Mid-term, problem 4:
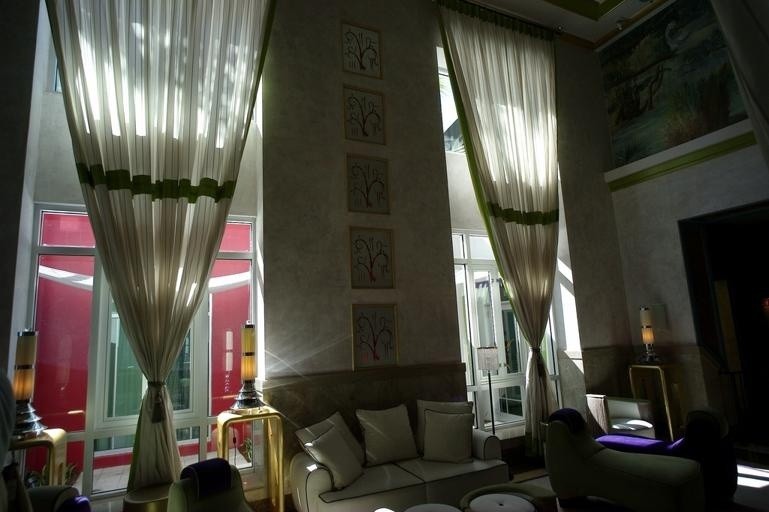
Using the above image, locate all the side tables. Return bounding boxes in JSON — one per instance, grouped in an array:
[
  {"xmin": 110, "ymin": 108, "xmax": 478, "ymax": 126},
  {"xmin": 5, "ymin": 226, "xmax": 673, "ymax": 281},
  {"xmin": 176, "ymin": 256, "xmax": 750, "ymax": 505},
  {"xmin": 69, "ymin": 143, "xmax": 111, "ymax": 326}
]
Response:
[
  {"xmin": 4, "ymin": 428, "xmax": 69, "ymax": 485},
  {"xmin": 217, "ymin": 404, "xmax": 286, "ymax": 512},
  {"xmin": 629, "ymin": 362, "xmax": 681, "ymax": 443}
]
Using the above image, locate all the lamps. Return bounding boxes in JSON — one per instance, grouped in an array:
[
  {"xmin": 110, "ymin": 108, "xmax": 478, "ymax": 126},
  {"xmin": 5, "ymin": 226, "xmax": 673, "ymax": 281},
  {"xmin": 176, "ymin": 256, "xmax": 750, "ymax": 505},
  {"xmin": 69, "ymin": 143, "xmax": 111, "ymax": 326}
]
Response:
[
  {"xmin": 229, "ymin": 324, "xmax": 265, "ymax": 411},
  {"xmin": 636, "ymin": 304, "xmax": 666, "ymax": 364},
  {"xmin": 10, "ymin": 328, "xmax": 48, "ymax": 434},
  {"xmin": 476, "ymin": 346, "xmax": 499, "ymax": 436}
]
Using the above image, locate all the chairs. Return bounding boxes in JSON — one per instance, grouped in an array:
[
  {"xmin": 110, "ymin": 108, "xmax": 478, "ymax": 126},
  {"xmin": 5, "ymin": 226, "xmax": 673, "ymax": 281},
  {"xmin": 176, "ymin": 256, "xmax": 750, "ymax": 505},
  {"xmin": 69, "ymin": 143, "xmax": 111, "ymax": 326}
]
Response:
[{"xmin": 168, "ymin": 458, "xmax": 255, "ymax": 512}]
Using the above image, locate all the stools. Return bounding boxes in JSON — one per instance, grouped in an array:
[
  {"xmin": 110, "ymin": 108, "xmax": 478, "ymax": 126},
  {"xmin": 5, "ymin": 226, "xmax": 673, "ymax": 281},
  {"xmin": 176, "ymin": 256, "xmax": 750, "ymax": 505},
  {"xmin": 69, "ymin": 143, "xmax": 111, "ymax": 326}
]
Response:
[
  {"xmin": 123, "ymin": 482, "xmax": 169, "ymax": 512},
  {"xmin": 403, "ymin": 483, "xmax": 558, "ymax": 512},
  {"xmin": 547, "ymin": 392, "xmax": 738, "ymax": 512}
]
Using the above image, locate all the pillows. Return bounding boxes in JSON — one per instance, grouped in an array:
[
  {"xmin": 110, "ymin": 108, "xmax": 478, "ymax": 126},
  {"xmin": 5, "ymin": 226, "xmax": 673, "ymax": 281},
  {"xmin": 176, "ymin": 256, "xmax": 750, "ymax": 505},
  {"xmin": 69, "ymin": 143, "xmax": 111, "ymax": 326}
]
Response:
[{"xmin": 294, "ymin": 399, "xmax": 474, "ymax": 490}]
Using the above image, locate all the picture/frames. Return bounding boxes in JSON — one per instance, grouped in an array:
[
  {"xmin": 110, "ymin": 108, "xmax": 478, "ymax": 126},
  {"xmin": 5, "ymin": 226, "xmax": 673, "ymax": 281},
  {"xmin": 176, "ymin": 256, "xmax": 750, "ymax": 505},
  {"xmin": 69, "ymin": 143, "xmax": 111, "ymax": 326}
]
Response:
[
  {"xmin": 351, "ymin": 304, "xmax": 400, "ymax": 372},
  {"xmin": 345, "ymin": 152, "xmax": 390, "ymax": 215},
  {"xmin": 340, "ymin": 21, "xmax": 384, "ymax": 81},
  {"xmin": 350, "ymin": 225, "xmax": 396, "ymax": 289},
  {"xmin": 342, "ymin": 84, "xmax": 387, "ymax": 147},
  {"xmin": 595, "ymin": 0, "xmax": 759, "ymax": 191}
]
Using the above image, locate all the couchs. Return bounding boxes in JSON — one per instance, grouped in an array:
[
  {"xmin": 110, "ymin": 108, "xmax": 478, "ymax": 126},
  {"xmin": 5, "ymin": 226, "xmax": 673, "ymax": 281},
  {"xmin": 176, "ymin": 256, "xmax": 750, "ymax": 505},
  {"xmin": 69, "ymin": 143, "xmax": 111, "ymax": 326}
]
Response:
[
  {"xmin": 7, "ymin": 486, "xmax": 91, "ymax": 512},
  {"xmin": 265, "ymin": 362, "xmax": 512, "ymax": 512}
]
